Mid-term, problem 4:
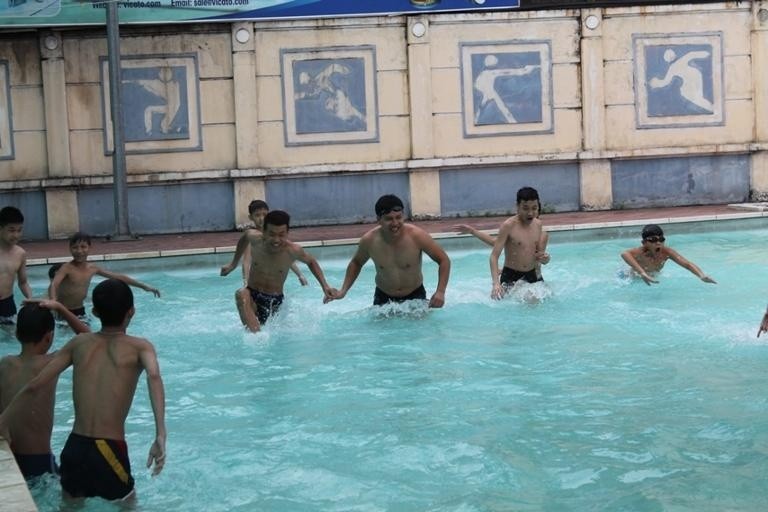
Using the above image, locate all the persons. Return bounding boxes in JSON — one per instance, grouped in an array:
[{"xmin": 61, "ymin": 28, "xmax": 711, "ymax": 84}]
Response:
[
  {"xmin": 241, "ymin": 198, "xmax": 309, "ymax": 289},
  {"xmin": 451, "ymin": 198, "xmax": 551, "ymax": 282},
  {"xmin": 47, "ymin": 262, "xmax": 63, "ymax": 281},
  {"xmin": 47, "ymin": 231, "xmax": 160, "ymax": 336},
  {"xmin": 0, "ymin": 205, "xmax": 33, "ymax": 343},
  {"xmin": 219, "ymin": 209, "xmax": 338, "ymax": 334},
  {"xmin": 0, "ymin": 298, "xmax": 94, "ymax": 487},
  {"xmin": 757, "ymin": 308, "xmax": 768, "ymax": 337},
  {"xmin": 487, "ymin": 186, "xmax": 552, "ymax": 303},
  {"xmin": 321, "ymin": 193, "xmax": 451, "ymax": 319},
  {"xmin": 617, "ymin": 223, "xmax": 718, "ymax": 287},
  {"xmin": 0, "ymin": 279, "xmax": 168, "ymax": 511}
]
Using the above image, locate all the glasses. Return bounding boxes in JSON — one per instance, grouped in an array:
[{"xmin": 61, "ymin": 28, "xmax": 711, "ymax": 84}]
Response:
[{"xmin": 644, "ymin": 236, "xmax": 665, "ymax": 243}]
